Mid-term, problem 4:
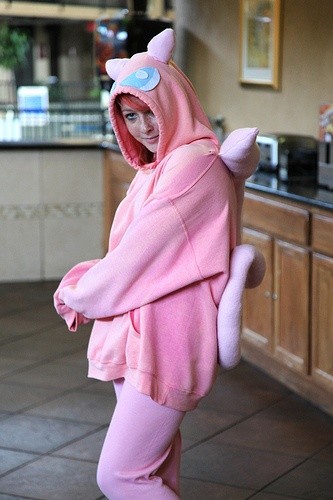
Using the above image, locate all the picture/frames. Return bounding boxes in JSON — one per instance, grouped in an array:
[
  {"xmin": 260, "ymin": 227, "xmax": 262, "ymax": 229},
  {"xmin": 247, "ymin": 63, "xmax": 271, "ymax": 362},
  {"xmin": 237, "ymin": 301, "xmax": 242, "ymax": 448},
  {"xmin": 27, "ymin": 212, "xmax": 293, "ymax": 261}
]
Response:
[{"xmin": 238, "ymin": 0, "xmax": 284, "ymax": 90}]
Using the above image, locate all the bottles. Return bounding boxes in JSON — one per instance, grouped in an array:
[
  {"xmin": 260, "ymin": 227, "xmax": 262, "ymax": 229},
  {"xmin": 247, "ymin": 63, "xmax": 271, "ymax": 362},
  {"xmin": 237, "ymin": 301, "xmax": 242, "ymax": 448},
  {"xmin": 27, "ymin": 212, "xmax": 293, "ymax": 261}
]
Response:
[{"xmin": 18, "ymin": 109, "xmax": 104, "ymax": 139}]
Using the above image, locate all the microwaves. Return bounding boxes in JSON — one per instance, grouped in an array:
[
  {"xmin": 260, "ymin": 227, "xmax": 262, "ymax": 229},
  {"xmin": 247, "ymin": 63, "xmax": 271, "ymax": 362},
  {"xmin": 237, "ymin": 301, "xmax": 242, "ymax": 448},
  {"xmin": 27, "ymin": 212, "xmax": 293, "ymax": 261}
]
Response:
[{"xmin": 256, "ymin": 132, "xmax": 319, "ymax": 183}]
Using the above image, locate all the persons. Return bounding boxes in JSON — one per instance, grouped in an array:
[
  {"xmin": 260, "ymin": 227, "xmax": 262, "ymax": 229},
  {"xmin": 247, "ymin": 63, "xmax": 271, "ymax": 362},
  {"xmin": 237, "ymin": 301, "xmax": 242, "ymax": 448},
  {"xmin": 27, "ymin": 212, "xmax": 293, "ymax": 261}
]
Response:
[{"xmin": 52, "ymin": 52, "xmax": 238, "ymax": 500}]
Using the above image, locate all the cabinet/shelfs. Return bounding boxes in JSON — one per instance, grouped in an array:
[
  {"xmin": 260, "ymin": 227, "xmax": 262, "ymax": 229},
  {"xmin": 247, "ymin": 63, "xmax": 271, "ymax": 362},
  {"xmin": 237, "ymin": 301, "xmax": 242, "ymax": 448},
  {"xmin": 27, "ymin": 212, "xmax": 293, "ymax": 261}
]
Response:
[{"xmin": 103, "ymin": 148, "xmax": 332, "ymax": 416}]
